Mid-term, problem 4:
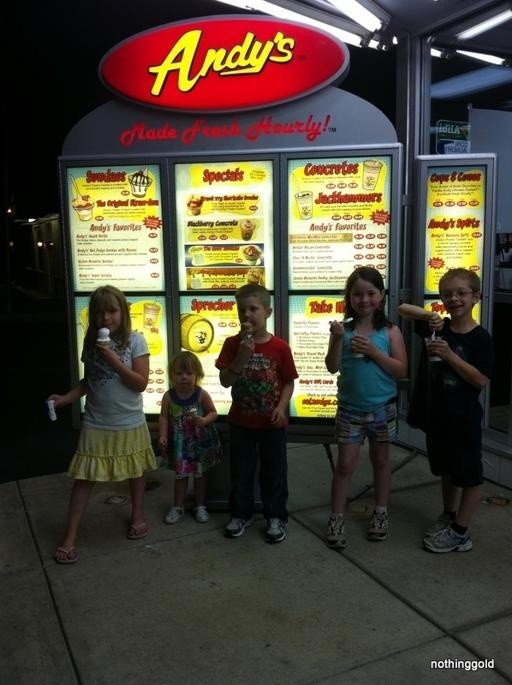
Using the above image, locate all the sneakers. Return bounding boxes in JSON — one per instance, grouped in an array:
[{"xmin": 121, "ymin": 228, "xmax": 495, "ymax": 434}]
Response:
[
  {"xmin": 423, "ymin": 524, "xmax": 474, "ymax": 553},
  {"xmin": 262, "ymin": 516, "xmax": 288, "ymax": 543},
  {"xmin": 324, "ymin": 516, "xmax": 348, "ymax": 548},
  {"xmin": 424, "ymin": 512, "xmax": 457, "ymax": 538},
  {"xmin": 223, "ymin": 514, "xmax": 255, "ymax": 538},
  {"xmin": 365, "ymin": 509, "xmax": 391, "ymax": 540}
]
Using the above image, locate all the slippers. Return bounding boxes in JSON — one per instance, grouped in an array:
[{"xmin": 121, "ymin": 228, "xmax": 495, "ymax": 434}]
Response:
[
  {"xmin": 127, "ymin": 519, "xmax": 151, "ymax": 539},
  {"xmin": 54, "ymin": 545, "xmax": 80, "ymax": 563}
]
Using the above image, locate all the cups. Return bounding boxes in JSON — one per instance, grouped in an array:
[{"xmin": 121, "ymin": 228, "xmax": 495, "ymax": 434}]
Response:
[
  {"xmin": 144, "ymin": 302, "xmax": 161, "ymax": 328},
  {"xmin": 128, "ymin": 176, "xmax": 152, "ymax": 198},
  {"xmin": 423, "ymin": 338, "xmax": 446, "ymax": 362},
  {"xmin": 350, "ymin": 337, "xmax": 366, "ymax": 359},
  {"xmin": 246, "ymin": 270, "xmax": 260, "ymax": 283},
  {"xmin": 295, "ymin": 191, "xmax": 315, "ymax": 219},
  {"xmin": 363, "ymin": 160, "xmax": 382, "ymax": 190},
  {"xmin": 187, "ymin": 201, "xmax": 204, "ymax": 214},
  {"xmin": 239, "ymin": 224, "xmax": 256, "ymax": 240},
  {"xmin": 244, "ymin": 244, "xmax": 262, "ymax": 266},
  {"xmin": 189, "ymin": 246, "xmax": 206, "ymax": 264}
]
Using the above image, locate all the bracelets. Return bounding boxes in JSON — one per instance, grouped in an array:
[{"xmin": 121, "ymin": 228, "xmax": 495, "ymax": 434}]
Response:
[{"xmin": 228, "ymin": 367, "xmax": 243, "ymax": 377}]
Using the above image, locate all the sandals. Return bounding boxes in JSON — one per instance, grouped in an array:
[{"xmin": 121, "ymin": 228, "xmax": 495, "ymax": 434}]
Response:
[
  {"xmin": 165, "ymin": 504, "xmax": 185, "ymax": 525},
  {"xmin": 193, "ymin": 504, "xmax": 210, "ymax": 523}
]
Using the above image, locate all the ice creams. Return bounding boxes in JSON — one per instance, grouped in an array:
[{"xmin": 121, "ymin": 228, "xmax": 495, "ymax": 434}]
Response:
[
  {"xmin": 247, "ymin": 269, "xmax": 260, "ymax": 285},
  {"xmin": 130, "ymin": 169, "xmax": 148, "ymax": 196},
  {"xmin": 241, "ymin": 220, "xmax": 254, "ymax": 240},
  {"xmin": 241, "ymin": 322, "xmax": 253, "ymax": 340},
  {"xmin": 188, "ymin": 198, "xmax": 203, "ymax": 215},
  {"xmin": 96, "ymin": 327, "xmax": 112, "ymax": 346},
  {"xmin": 243, "ymin": 246, "xmax": 260, "ymax": 265}
]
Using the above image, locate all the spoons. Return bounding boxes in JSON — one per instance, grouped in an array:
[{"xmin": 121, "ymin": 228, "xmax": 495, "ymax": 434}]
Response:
[
  {"xmin": 329, "ymin": 317, "xmax": 352, "ymax": 324},
  {"xmin": 431, "ymin": 324, "xmax": 436, "ymax": 341}
]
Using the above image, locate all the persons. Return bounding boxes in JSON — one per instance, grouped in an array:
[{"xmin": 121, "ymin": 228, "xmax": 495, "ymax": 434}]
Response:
[
  {"xmin": 396, "ymin": 265, "xmax": 495, "ymax": 555},
  {"xmin": 46, "ymin": 284, "xmax": 155, "ymax": 564},
  {"xmin": 215, "ymin": 283, "xmax": 298, "ymax": 544},
  {"xmin": 155, "ymin": 351, "xmax": 225, "ymax": 527},
  {"xmin": 323, "ymin": 265, "xmax": 408, "ymax": 551}
]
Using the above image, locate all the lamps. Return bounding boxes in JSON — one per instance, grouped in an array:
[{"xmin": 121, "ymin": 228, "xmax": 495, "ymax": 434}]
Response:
[
  {"xmin": 218, "ymin": 0, "xmax": 399, "ymax": 55},
  {"xmin": 431, "ymin": 5, "xmax": 512, "ymax": 67}
]
